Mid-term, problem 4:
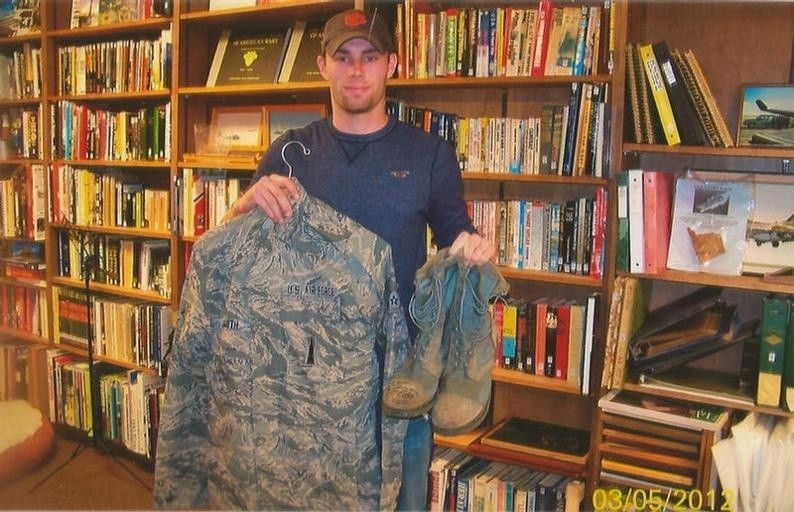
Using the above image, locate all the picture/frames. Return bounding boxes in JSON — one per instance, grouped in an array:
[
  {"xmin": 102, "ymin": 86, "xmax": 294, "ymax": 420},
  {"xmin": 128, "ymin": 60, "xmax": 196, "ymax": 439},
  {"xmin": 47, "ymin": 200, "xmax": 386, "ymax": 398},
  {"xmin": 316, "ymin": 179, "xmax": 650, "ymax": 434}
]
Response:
[
  {"xmin": 680, "ymin": 165, "xmax": 794, "ymax": 274},
  {"xmin": 736, "ymin": 81, "xmax": 788, "ymax": 147},
  {"xmin": 268, "ymin": 102, "xmax": 326, "ymax": 147},
  {"xmin": 209, "ymin": 103, "xmax": 266, "ymax": 154}
]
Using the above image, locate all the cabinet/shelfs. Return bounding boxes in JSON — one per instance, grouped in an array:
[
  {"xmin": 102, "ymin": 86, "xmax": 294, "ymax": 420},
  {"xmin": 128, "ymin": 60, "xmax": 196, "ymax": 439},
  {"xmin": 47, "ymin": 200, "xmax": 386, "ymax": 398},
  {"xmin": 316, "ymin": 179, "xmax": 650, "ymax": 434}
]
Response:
[{"xmin": 1, "ymin": 0, "xmax": 786, "ymax": 510}]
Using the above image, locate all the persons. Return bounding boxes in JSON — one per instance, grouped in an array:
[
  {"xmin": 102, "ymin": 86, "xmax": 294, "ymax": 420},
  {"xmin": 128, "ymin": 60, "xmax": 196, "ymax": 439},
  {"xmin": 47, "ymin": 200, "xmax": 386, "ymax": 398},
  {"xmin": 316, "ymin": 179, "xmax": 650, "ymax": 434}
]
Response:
[{"xmin": 218, "ymin": 8, "xmax": 496, "ymax": 512}]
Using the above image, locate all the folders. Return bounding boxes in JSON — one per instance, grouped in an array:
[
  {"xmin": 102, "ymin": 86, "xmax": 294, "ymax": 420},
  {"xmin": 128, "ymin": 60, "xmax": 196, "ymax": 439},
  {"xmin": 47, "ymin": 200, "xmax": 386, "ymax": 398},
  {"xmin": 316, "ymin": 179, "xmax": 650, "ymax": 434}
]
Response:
[
  {"xmin": 639, "ymin": 44, "xmax": 681, "ymax": 146},
  {"xmin": 628, "ymin": 284, "xmax": 736, "ymax": 367},
  {"xmin": 651, "ymin": 37, "xmax": 700, "ymax": 142},
  {"xmin": 779, "ymin": 296, "xmax": 794, "ymax": 412},
  {"xmin": 756, "ymin": 294, "xmax": 790, "ymax": 407},
  {"xmin": 632, "ymin": 319, "xmax": 760, "ymax": 377},
  {"xmin": 615, "ymin": 168, "xmax": 631, "ymax": 276},
  {"xmin": 642, "ymin": 171, "xmax": 674, "ymax": 275},
  {"xmin": 628, "ymin": 168, "xmax": 645, "ymax": 273}
]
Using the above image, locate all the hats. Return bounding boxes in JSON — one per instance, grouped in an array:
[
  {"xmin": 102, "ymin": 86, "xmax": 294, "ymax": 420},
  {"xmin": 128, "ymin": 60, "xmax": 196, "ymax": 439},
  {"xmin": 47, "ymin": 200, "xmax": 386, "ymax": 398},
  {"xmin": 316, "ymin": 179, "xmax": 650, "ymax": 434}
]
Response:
[{"xmin": 321, "ymin": 10, "xmax": 391, "ymax": 56}]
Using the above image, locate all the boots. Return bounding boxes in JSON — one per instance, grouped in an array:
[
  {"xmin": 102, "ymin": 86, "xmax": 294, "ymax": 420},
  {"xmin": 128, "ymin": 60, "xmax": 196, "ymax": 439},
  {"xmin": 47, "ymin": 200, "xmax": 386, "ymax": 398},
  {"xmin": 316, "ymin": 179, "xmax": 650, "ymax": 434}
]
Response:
[
  {"xmin": 429, "ymin": 260, "xmax": 511, "ymax": 436},
  {"xmin": 382, "ymin": 248, "xmax": 457, "ymax": 418}
]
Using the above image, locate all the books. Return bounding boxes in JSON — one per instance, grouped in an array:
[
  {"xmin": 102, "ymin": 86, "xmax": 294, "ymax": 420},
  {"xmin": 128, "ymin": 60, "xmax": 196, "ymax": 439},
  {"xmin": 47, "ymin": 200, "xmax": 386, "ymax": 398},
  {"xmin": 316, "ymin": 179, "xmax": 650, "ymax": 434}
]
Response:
[
  {"xmin": 425, "ymin": 186, "xmax": 610, "ymax": 280},
  {"xmin": 426, "ymin": 442, "xmax": 587, "ymax": 512},
  {"xmin": 488, "ymin": 290, "xmax": 601, "ymax": 396},
  {"xmin": 624, "ymin": 40, "xmax": 736, "ymax": 148},
  {"xmin": 176, "ymin": 167, "xmax": 252, "ymax": 239},
  {"xmin": 70, "ymin": 0, "xmax": 160, "ymax": 31},
  {"xmin": 49, "ymin": 99, "xmax": 172, "ymax": 162},
  {"xmin": 384, "ymin": 80, "xmax": 613, "ymax": 179},
  {"xmin": 214, "ymin": 25, "xmax": 294, "ymax": 86},
  {"xmin": 0, "ymin": 0, "xmax": 41, "ymax": 38},
  {"xmin": 0, "ymin": 283, "xmax": 50, "ymax": 339},
  {"xmin": 183, "ymin": 147, "xmax": 261, "ymax": 168},
  {"xmin": 596, "ymin": 390, "xmax": 794, "ymax": 512},
  {"xmin": 0, "ymin": 42, "xmax": 43, "ymax": 101},
  {"xmin": 600, "ymin": 275, "xmax": 794, "ymax": 415},
  {"xmin": 0, "ymin": 342, "xmax": 165, "ymax": 462},
  {"xmin": 288, "ymin": 19, "xmax": 327, "ymax": 82},
  {"xmin": 0, "ymin": 104, "xmax": 44, "ymax": 162},
  {"xmin": 47, "ymin": 163, "xmax": 173, "ymax": 235},
  {"xmin": 616, "ymin": 168, "xmax": 675, "ymax": 274},
  {"xmin": 468, "ymin": 413, "xmax": 591, "ymax": 473},
  {"xmin": 393, "ymin": 0, "xmax": 616, "ymax": 79},
  {"xmin": 1, "ymin": 240, "xmax": 47, "ymax": 287},
  {"xmin": 56, "ymin": 228, "xmax": 174, "ymax": 301},
  {"xmin": 184, "ymin": 241, "xmax": 194, "ymax": 272},
  {"xmin": 56, "ymin": 29, "xmax": 174, "ymax": 95},
  {"xmin": 51, "ymin": 284, "xmax": 174, "ymax": 378},
  {"xmin": 0, "ymin": 163, "xmax": 46, "ymax": 241}
]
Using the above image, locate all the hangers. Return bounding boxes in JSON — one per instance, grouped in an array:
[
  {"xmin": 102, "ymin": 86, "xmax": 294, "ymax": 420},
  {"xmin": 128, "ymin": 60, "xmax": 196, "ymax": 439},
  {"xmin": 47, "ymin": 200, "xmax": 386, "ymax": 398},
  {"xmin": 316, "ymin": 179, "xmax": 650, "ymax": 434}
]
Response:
[{"xmin": 264, "ymin": 140, "xmax": 312, "ymax": 202}]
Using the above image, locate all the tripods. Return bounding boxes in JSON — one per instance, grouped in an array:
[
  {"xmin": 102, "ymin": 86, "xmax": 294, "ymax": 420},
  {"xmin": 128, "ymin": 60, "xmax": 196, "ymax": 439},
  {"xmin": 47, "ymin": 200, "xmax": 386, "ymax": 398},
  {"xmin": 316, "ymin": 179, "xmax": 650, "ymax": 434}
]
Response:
[{"xmin": 27, "ymin": 210, "xmax": 153, "ymax": 495}]
